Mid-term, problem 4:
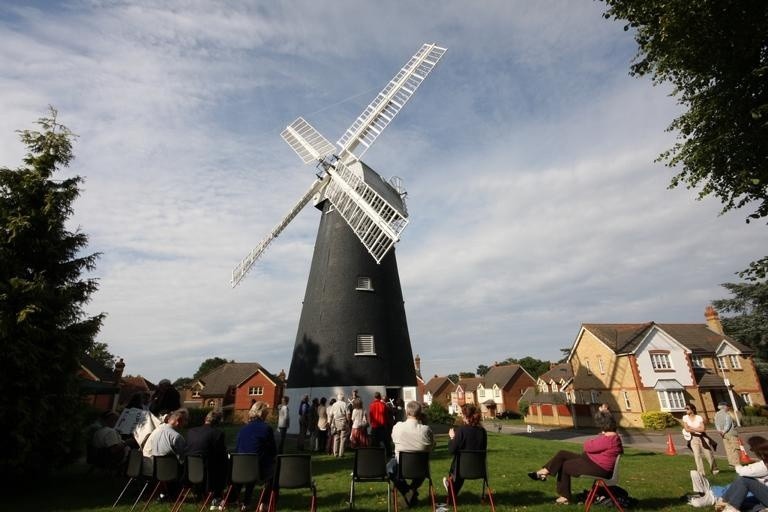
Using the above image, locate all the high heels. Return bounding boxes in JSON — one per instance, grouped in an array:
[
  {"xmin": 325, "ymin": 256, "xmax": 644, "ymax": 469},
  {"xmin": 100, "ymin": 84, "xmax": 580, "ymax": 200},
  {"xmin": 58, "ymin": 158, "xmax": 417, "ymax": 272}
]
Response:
[
  {"xmin": 664, "ymin": 434, "xmax": 678, "ymax": 458},
  {"xmin": 734, "ymin": 438, "xmax": 756, "ymax": 468}
]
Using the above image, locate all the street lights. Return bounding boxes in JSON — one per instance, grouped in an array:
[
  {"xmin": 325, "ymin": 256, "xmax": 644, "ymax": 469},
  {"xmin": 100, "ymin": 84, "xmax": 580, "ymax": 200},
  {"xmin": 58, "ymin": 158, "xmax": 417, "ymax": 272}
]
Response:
[{"xmin": 361, "ymin": 416, "xmax": 368, "ymax": 427}]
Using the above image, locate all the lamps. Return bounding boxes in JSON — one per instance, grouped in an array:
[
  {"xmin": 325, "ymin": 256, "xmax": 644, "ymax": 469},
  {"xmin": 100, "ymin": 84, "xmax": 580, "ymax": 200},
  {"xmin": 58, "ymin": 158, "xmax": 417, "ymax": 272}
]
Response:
[
  {"xmin": 110, "ymin": 449, "xmax": 317, "ymax": 511},
  {"xmin": 348, "ymin": 446, "xmax": 495, "ymax": 511},
  {"xmin": 579, "ymin": 455, "xmax": 622, "ymax": 509}
]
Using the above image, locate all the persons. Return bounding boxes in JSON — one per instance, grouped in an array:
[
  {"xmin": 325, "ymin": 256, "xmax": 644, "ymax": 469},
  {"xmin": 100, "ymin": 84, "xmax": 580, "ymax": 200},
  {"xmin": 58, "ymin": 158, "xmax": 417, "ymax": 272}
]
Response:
[
  {"xmin": 598, "ymin": 403, "xmax": 613, "ymax": 417},
  {"xmin": 94, "ymin": 411, "xmax": 134, "ymax": 464},
  {"xmin": 713, "ymin": 435, "xmax": 767, "ymax": 512},
  {"xmin": 443, "ymin": 403, "xmax": 487, "ymax": 509},
  {"xmin": 386, "ymin": 400, "xmax": 436, "ymax": 509},
  {"xmin": 680, "ymin": 404, "xmax": 719, "ymax": 478},
  {"xmin": 276, "ymin": 396, "xmax": 292, "ymax": 454},
  {"xmin": 713, "ymin": 401, "xmax": 742, "ymax": 472},
  {"xmin": 150, "ymin": 409, "xmax": 189, "ymax": 502},
  {"xmin": 233, "ymin": 401, "xmax": 277, "ymax": 499},
  {"xmin": 298, "ymin": 388, "xmax": 406, "ymax": 458},
  {"xmin": 527, "ymin": 412, "xmax": 624, "ymax": 506},
  {"xmin": 185, "ymin": 410, "xmax": 233, "ymax": 509}
]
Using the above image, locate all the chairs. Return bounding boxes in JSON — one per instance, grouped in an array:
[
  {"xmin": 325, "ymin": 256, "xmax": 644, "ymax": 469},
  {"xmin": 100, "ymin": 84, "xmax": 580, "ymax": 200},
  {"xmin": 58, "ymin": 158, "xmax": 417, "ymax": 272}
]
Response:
[
  {"xmin": 528, "ymin": 472, "xmax": 546, "ymax": 481},
  {"xmin": 210, "ymin": 497, "xmax": 221, "ymax": 510},
  {"xmin": 219, "ymin": 499, "xmax": 228, "ymax": 510},
  {"xmin": 443, "ymin": 477, "xmax": 449, "ymax": 490}
]
